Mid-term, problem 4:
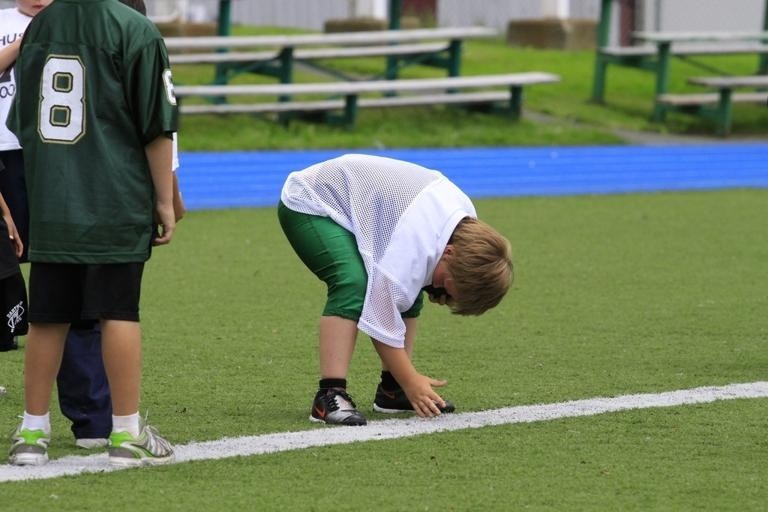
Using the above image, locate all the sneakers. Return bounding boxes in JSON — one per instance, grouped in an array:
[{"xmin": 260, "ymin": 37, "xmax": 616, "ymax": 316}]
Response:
[
  {"xmin": 309, "ymin": 386, "xmax": 367, "ymax": 427},
  {"xmin": 75, "ymin": 434, "xmax": 111, "ymax": 449},
  {"xmin": 7, "ymin": 427, "xmax": 52, "ymax": 466},
  {"xmin": 107, "ymin": 423, "xmax": 178, "ymax": 465},
  {"xmin": 371, "ymin": 382, "xmax": 455, "ymax": 414}
]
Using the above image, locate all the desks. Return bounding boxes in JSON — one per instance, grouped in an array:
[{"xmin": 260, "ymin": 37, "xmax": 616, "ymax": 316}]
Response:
[
  {"xmin": 632, "ymin": 31, "xmax": 767, "ymax": 126},
  {"xmin": 162, "ymin": 26, "xmax": 500, "ymax": 129}
]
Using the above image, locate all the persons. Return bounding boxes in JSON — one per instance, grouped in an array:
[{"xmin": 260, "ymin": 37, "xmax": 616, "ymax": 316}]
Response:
[
  {"xmin": 277, "ymin": 153, "xmax": 515, "ymax": 426},
  {"xmin": 0, "ymin": 1, "xmax": 186, "ymax": 467}
]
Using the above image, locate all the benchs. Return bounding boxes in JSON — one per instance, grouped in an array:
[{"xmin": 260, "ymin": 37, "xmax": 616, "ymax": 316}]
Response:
[
  {"xmin": 590, "ymin": 42, "xmax": 767, "ymax": 124},
  {"xmin": 161, "ymin": 42, "xmax": 562, "ymax": 130}
]
